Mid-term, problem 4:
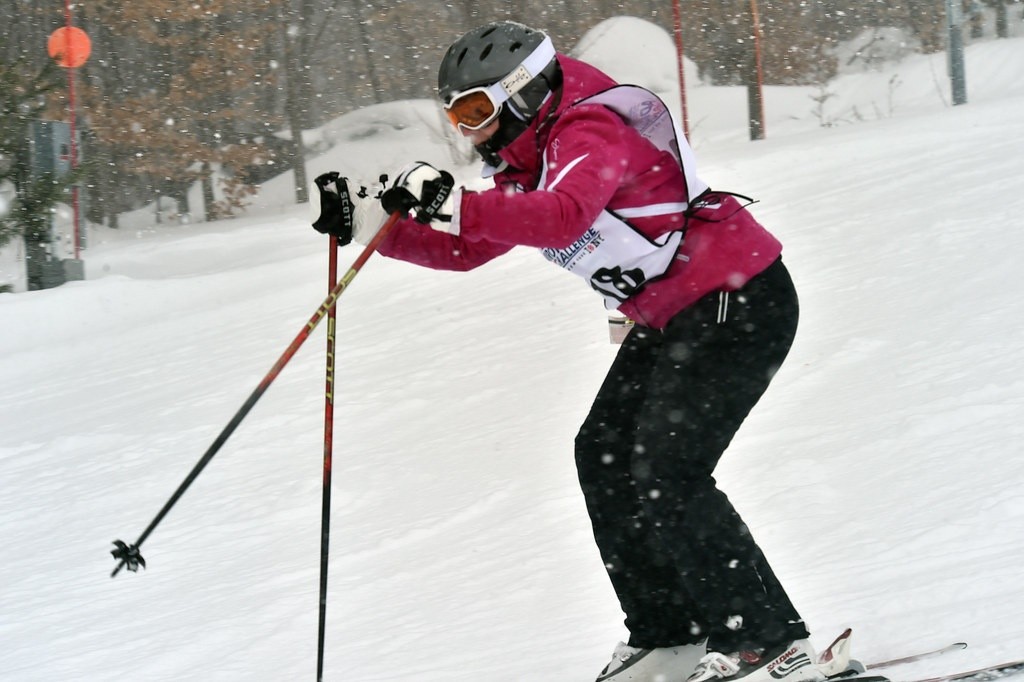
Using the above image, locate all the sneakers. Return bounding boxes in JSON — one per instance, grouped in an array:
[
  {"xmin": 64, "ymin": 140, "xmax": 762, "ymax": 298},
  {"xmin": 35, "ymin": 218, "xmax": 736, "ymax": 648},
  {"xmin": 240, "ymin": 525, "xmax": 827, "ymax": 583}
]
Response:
[
  {"xmin": 687, "ymin": 639, "xmax": 826, "ymax": 682},
  {"xmin": 595, "ymin": 634, "xmax": 708, "ymax": 681}
]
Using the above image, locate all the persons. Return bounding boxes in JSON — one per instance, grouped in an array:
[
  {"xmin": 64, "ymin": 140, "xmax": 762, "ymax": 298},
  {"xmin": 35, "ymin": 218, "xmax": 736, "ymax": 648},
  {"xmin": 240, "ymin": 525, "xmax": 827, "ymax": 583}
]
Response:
[{"xmin": 314, "ymin": 19, "xmax": 823, "ymax": 682}]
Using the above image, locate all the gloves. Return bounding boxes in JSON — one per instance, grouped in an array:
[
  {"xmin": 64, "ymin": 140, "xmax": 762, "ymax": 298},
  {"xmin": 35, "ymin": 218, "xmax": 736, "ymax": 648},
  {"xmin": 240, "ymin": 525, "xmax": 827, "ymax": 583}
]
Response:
[
  {"xmin": 381, "ymin": 160, "xmax": 465, "ymax": 238},
  {"xmin": 308, "ymin": 170, "xmax": 391, "ymax": 247}
]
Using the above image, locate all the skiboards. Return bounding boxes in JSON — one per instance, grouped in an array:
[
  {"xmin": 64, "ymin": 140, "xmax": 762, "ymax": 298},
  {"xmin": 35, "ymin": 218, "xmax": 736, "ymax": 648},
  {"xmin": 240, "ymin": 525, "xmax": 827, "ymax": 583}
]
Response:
[{"xmin": 594, "ymin": 640, "xmax": 1023, "ymax": 682}]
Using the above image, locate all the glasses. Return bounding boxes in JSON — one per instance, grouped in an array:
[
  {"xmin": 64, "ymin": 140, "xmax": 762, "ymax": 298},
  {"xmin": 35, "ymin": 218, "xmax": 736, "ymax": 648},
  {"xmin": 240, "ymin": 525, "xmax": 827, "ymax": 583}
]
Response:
[{"xmin": 443, "ymin": 36, "xmax": 556, "ymax": 138}]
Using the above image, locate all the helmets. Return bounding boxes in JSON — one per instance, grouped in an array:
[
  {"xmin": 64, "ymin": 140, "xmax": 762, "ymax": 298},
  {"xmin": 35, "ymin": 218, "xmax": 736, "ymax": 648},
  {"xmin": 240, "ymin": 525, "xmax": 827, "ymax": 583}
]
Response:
[{"xmin": 436, "ymin": 19, "xmax": 564, "ymax": 173}]
